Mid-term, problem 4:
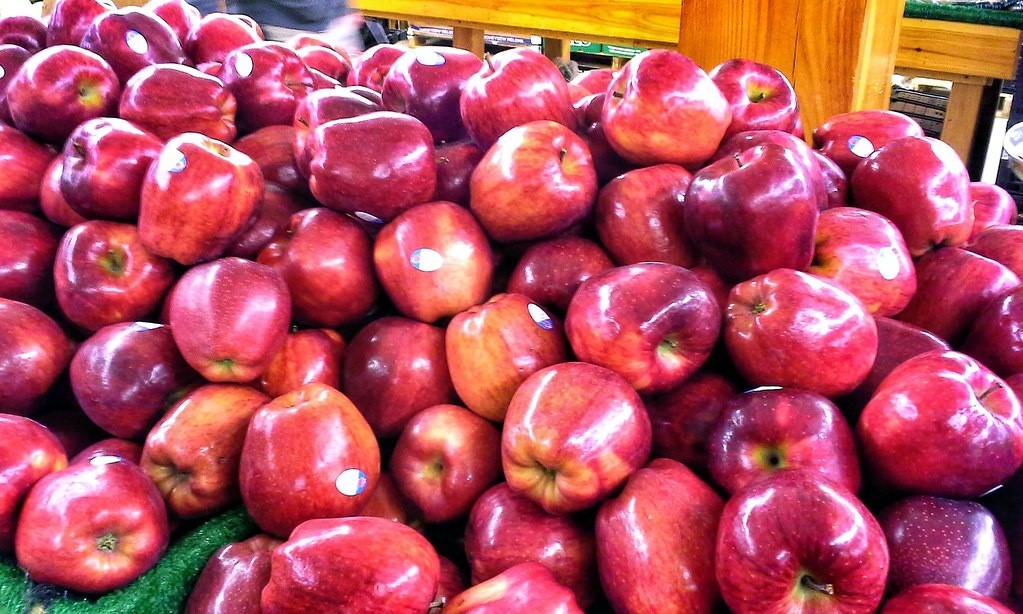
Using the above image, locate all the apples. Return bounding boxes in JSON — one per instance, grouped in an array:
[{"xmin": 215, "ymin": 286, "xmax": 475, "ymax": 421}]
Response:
[{"xmin": 0, "ymin": 0, "xmax": 1023, "ymax": 614}]
